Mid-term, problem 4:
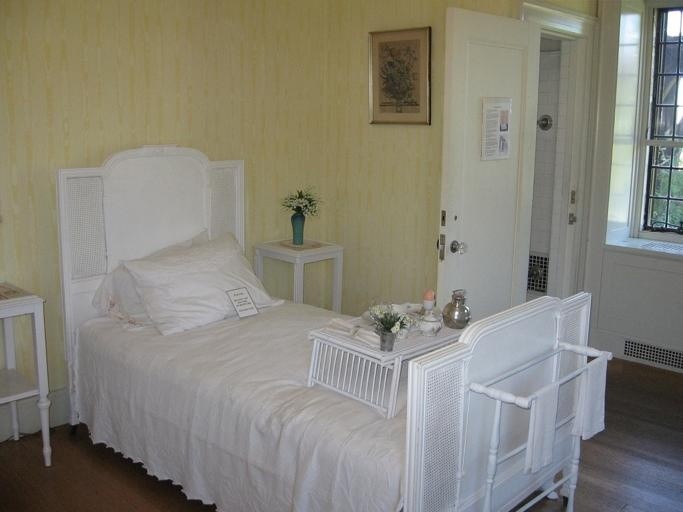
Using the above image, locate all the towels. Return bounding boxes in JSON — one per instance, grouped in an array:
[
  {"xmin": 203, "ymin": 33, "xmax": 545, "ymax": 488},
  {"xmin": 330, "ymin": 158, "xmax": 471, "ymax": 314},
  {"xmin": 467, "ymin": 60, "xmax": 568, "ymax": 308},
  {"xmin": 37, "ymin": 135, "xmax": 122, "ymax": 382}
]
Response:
[
  {"xmin": 569, "ymin": 354, "xmax": 606, "ymax": 440},
  {"xmin": 523, "ymin": 382, "xmax": 559, "ymax": 474}
]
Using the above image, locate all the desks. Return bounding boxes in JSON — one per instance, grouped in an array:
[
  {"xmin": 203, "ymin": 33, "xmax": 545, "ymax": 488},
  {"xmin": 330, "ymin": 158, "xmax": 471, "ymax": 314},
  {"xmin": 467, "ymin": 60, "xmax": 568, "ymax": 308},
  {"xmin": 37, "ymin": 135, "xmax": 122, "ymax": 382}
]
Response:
[{"xmin": 306, "ymin": 304, "xmax": 469, "ymax": 418}]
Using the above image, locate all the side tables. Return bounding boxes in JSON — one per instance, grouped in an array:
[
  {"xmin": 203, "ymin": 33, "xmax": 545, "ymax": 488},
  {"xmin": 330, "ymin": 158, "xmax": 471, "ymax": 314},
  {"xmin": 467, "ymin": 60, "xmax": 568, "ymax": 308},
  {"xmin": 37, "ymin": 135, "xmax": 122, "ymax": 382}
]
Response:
[
  {"xmin": 0, "ymin": 282, "xmax": 52, "ymax": 469},
  {"xmin": 255, "ymin": 238, "xmax": 345, "ymax": 308}
]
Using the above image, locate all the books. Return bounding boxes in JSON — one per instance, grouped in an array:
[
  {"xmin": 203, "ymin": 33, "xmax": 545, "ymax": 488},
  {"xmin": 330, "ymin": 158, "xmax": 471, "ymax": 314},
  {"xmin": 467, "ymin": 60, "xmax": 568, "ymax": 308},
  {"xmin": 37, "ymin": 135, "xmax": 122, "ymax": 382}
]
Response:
[{"xmin": 224, "ymin": 286, "xmax": 260, "ymax": 322}]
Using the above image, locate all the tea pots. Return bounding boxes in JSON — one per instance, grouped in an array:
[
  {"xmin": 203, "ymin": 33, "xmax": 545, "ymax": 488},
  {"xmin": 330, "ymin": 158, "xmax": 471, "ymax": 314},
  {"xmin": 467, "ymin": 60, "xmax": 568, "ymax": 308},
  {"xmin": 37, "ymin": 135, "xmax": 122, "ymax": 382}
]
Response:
[
  {"xmin": 443, "ymin": 289, "xmax": 472, "ymax": 329},
  {"xmin": 414, "ymin": 310, "xmax": 443, "ymax": 337}
]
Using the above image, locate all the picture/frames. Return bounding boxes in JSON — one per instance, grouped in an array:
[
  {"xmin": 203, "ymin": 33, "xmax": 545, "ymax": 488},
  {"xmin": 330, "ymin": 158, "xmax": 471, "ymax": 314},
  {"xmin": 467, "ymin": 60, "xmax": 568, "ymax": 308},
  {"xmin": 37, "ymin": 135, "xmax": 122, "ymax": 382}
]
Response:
[{"xmin": 366, "ymin": 26, "xmax": 432, "ymax": 125}]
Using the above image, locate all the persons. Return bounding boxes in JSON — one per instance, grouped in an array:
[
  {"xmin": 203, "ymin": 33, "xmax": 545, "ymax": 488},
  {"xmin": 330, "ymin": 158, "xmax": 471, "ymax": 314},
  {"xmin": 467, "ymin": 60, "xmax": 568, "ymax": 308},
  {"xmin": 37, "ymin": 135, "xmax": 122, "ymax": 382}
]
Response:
[
  {"xmin": 502, "ymin": 139, "xmax": 508, "ymax": 155},
  {"xmin": 499, "ymin": 111, "xmax": 508, "ymax": 131}
]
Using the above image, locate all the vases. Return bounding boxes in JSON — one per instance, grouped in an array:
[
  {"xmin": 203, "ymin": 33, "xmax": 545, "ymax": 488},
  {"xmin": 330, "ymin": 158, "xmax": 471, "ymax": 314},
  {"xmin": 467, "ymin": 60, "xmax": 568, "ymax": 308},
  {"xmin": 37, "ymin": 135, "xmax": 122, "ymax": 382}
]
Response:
[
  {"xmin": 380, "ymin": 330, "xmax": 394, "ymax": 350},
  {"xmin": 291, "ymin": 211, "xmax": 305, "ymax": 246}
]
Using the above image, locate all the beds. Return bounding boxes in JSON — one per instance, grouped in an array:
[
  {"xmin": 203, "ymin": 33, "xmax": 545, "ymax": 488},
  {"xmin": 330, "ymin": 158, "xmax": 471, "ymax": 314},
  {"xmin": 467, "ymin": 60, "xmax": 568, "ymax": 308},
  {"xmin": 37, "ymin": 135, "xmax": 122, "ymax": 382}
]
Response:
[{"xmin": 58, "ymin": 144, "xmax": 592, "ymax": 512}]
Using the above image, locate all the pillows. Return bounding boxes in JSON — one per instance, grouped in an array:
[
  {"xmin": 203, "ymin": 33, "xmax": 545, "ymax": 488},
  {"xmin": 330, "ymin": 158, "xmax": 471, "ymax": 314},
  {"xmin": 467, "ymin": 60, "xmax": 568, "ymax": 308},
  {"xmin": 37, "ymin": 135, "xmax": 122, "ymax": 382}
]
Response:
[
  {"xmin": 98, "ymin": 230, "xmax": 212, "ymax": 328},
  {"xmin": 123, "ymin": 232, "xmax": 276, "ymax": 338}
]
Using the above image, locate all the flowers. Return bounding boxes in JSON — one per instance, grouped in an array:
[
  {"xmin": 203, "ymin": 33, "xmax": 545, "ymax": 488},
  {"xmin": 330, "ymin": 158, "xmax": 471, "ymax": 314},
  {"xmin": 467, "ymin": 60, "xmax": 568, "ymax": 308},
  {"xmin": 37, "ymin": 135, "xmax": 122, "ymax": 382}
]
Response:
[
  {"xmin": 283, "ymin": 183, "xmax": 323, "ymax": 215},
  {"xmin": 364, "ymin": 300, "xmax": 419, "ymax": 334}
]
